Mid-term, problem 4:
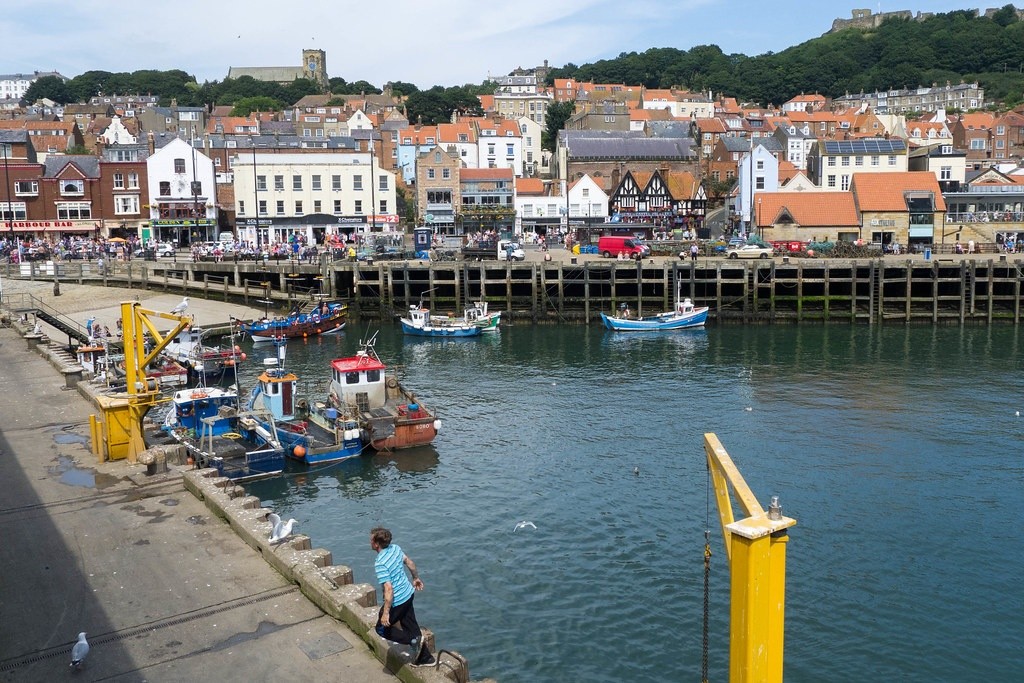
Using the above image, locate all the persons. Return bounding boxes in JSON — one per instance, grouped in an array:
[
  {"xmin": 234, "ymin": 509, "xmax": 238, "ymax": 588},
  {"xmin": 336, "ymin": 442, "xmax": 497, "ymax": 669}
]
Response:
[
  {"xmin": 1003, "ymin": 239, "xmax": 1024, "ymax": 254},
  {"xmin": 518, "ymin": 229, "xmax": 747, "ymax": 260},
  {"xmin": 467, "ymin": 229, "xmax": 502, "ymax": 248},
  {"xmin": 954, "ymin": 240, "xmax": 982, "ymax": 254},
  {"xmin": 191, "ymin": 232, "xmax": 404, "ymax": 262},
  {"xmin": 968, "ymin": 209, "xmax": 1020, "ymax": 222},
  {"xmin": 370, "ymin": 528, "xmax": 436, "ymax": 667},
  {"xmin": 0, "ymin": 233, "xmax": 173, "ymax": 269},
  {"xmin": 87, "ymin": 316, "xmax": 112, "ymax": 338},
  {"xmin": 886, "ymin": 241, "xmax": 900, "ymax": 255},
  {"xmin": 322, "ymin": 302, "xmax": 329, "ymax": 316}
]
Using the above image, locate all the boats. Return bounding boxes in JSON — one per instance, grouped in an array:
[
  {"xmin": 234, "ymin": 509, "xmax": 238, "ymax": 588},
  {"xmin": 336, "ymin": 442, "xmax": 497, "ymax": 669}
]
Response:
[
  {"xmin": 234, "ymin": 293, "xmax": 349, "ymax": 344},
  {"xmin": 237, "ymin": 316, "xmax": 366, "ymax": 467},
  {"xmin": 75, "ymin": 309, "xmax": 247, "ymax": 396},
  {"xmin": 161, "ymin": 322, "xmax": 288, "ymax": 486},
  {"xmin": 399, "ymin": 287, "xmax": 503, "ymax": 338},
  {"xmin": 311, "ymin": 318, "xmax": 442, "ymax": 454},
  {"xmin": 599, "ymin": 272, "xmax": 710, "ymax": 333}
]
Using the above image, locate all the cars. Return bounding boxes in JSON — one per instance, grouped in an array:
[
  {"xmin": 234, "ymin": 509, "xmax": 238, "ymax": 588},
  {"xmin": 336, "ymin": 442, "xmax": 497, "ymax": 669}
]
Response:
[
  {"xmin": 134, "ymin": 243, "xmax": 176, "ymax": 258},
  {"xmin": 726, "ymin": 243, "xmax": 774, "ymax": 260},
  {"xmin": 198, "ymin": 231, "xmax": 256, "ymax": 262},
  {"xmin": 61, "ymin": 245, "xmax": 100, "ymax": 260}
]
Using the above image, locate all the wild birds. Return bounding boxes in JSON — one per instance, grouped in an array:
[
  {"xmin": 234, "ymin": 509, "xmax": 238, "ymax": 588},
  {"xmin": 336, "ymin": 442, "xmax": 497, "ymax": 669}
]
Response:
[
  {"xmin": 68, "ymin": 631, "xmax": 90, "ymax": 667},
  {"xmin": 170, "ymin": 297, "xmax": 190, "ymax": 315},
  {"xmin": 513, "ymin": 520, "xmax": 536, "ymax": 533},
  {"xmin": 17, "ymin": 314, "xmax": 26, "ymax": 323},
  {"xmin": 745, "ymin": 406, "xmax": 752, "ymax": 412},
  {"xmin": 265, "ymin": 511, "xmax": 298, "ymax": 545},
  {"xmin": 1015, "ymin": 411, "xmax": 1020, "ymax": 417},
  {"xmin": 33, "ymin": 324, "xmax": 40, "ymax": 335},
  {"xmin": 634, "ymin": 466, "xmax": 639, "ymax": 475}
]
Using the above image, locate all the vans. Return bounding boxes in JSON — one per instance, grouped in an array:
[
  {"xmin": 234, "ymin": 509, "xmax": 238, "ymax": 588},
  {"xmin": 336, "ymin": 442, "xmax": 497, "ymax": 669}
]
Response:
[{"xmin": 598, "ymin": 236, "xmax": 650, "ymax": 260}]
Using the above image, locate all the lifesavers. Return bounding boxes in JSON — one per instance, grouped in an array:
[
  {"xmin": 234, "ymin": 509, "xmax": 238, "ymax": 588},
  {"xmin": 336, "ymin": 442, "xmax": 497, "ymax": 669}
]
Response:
[
  {"xmin": 190, "ymin": 393, "xmax": 207, "ymax": 399},
  {"xmin": 334, "ymin": 308, "xmax": 340, "ymax": 317},
  {"xmin": 312, "ymin": 314, "xmax": 320, "ymax": 323}
]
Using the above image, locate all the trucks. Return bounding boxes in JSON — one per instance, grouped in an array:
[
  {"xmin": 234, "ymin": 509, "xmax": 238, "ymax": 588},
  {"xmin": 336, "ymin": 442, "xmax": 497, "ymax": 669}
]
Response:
[{"xmin": 461, "ymin": 240, "xmax": 526, "ymax": 261}]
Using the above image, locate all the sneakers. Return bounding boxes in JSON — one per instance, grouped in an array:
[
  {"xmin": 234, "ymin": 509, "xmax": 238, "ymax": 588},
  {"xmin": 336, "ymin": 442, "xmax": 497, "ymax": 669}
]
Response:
[
  {"xmin": 410, "ymin": 635, "xmax": 424, "ymax": 663},
  {"xmin": 410, "ymin": 656, "xmax": 437, "ymax": 668}
]
